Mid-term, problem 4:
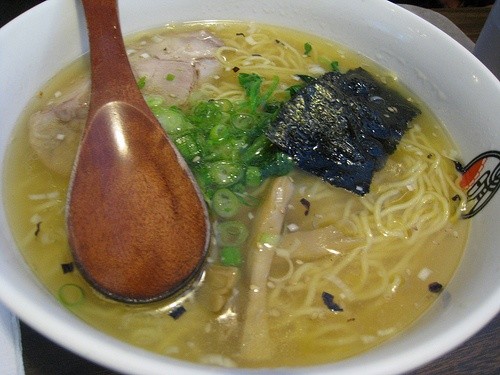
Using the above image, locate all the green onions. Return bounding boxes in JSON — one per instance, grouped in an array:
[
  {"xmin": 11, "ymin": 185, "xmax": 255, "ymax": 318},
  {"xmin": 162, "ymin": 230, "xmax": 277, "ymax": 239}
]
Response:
[{"xmin": 60, "ymin": 69, "xmax": 301, "ymax": 306}]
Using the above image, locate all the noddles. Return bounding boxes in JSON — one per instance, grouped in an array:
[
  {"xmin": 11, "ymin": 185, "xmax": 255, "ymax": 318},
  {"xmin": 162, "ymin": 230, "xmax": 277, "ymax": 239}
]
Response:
[{"xmin": 175, "ymin": 31, "xmax": 469, "ymax": 344}]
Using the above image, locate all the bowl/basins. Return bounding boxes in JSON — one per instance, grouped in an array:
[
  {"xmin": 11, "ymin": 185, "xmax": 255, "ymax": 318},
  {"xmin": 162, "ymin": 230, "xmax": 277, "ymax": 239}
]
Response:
[{"xmin": 0, "ymin": 0, "xmax": 499, "ymax": 375}]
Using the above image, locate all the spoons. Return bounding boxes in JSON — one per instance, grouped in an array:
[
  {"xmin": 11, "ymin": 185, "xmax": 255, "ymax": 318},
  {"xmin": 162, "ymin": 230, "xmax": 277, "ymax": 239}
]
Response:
[{"xmin": 65, "ymin": 0, "xmax": 211, "ymax": 303}]
